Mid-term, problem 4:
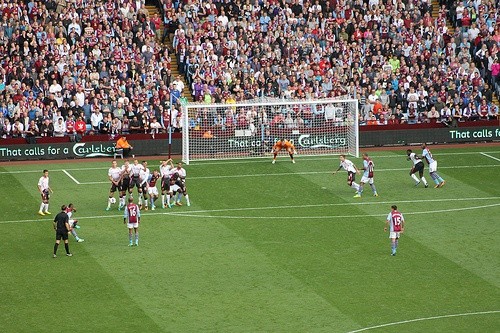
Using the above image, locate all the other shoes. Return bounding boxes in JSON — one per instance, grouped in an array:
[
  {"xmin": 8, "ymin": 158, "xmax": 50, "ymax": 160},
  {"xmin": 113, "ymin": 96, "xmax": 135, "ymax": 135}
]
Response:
[
  {"xmin": 135, "ymin": 243, "xmax": 139, "ymax": 246},
  {"xmin": 425, "ymin": 183, "xmax": 429, "ymax": 188},
  {"xmin": 139, "ymin": 205, "xmax": 142, "ymax": 210},
  {"xmin": 145, "ymin": 206, "xmax": 149, "ymax": 211},
  {"xmin": 53, "ymin": 254, "xmax": 57, "ymax": 258},
  {"xmin": 272, "ymin": 159, "xmax": 276, "ymax": 164},
  {"xmin": 440, "ymin": 180, "xmax": 446, "ymax": 188},
  {"xmin": 127, "ymin": 243, "xmax": 133, "ymax": 247},
  {"xmin": 38, "ymin": 211, "xmax": 45, "ymax": 216},
  {"xmin": 434, "ymin": 183, "xmax": 440, "ymax": 188},
  {"xmin": 150, "ymin": 203, "xmax": 156, "ymax": 210},
  {"xmin": 162, "ymin": 202, "xmax": 190, "ymax": 208},
  {"xmin": 391, "ymin": 248, "xmax": 397, "ymax": 255},
  {"xmin": 291, "ymin": 160, "xmax": 295, "ymax": 163},
  {"xmin": 77, "ymin": 239, "xmax": 84, "ymax": 243},
  {"xmin": 45, "ymin": 211, "xmax": 52, "ymax": 214},
  {"xmin": 66, "ymin": 254, "xmax": 72, "ymax": 256},
  {"xmin": 373, "ymin": 193, "xmax": 378, "ymax": 196},
  {"xmin": 355, "ymin": 190, "xmax": 363, "ymax": 193},
  {"xmin": 106, "ymin": 207, "xmax": 111, "ymax": 211},
  {"xmin": 415, "ymin": 180, "xmax": 420, "ymax": 186},
  {"xmin": 353, "ymin": 194, "xmax": 361, "ymax": 198}
]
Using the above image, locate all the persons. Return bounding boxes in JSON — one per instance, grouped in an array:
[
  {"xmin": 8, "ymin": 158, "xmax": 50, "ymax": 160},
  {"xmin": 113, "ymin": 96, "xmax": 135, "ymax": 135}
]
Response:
[
  {"xmin": 124, "ymin": 197, "xmax": 141, "ymax": 247},
  {"xmin": 52, "ymin": 205, "xmax": 73, "ymax": 258},
  {"xmin": 66, "ymin": 203, "xmax": 84, "ymax": 243},
  {"xmin": 0, "ymin": 0, "xmax": 500, "ymax": 216},
  {"xmin": 384, "ymin": 205, "xmax": 405, "ymax": 256}
]
